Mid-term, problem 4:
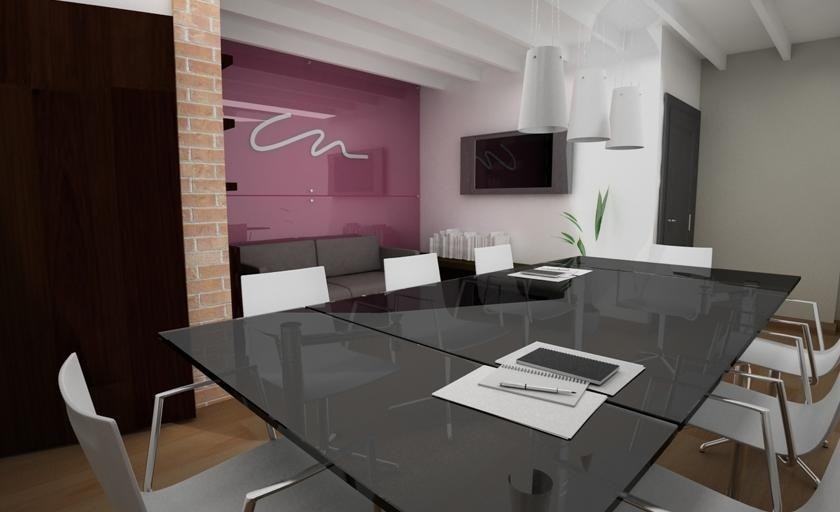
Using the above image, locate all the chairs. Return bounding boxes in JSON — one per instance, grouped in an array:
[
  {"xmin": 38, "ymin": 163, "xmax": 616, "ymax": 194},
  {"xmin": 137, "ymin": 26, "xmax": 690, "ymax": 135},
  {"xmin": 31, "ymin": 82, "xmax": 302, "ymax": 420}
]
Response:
[
  {"xmin": 240, "ymin": 264, "xmax": 335, "ymax": 441},
  {"xmin": 616, "ymin": 440, "xmax": 840, "ymax": 511},
  {"xmin": 56, "ymin": 350, "xmax": 372, "ymax": 508},
  {"xmin": 697, "ymin": 326, "xmax": 837, "ymax": 499},
  {"xmin": 735, "ymin": 284, "xmax": 838, "ymax": 398},
  {"xmin": 379, "ymin": 251, "xmax": 444, "ymax": 291},
  {"xmin": 647, "ymin": 243, "xmax": 714, "ymax": 277},
  {"xmin": 472, "ymin": 242, "xmax": 517, "ymax": 277}
]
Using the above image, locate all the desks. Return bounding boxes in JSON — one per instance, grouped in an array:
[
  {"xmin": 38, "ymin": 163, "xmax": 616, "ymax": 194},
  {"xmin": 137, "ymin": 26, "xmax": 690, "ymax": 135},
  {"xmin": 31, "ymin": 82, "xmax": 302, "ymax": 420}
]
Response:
[{"xmin": 168, "ymin": 255, "xmax": 803, "ymax": 511}]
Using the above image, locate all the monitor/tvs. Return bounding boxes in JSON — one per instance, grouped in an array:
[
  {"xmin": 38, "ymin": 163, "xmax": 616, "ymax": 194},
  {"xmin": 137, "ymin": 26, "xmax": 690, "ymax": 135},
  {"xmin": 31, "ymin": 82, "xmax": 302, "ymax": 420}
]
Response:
[
  {"xmin": 460, "ymin": 130, "xmax": 572, "ymax": 194},
  {"xmin": 326, "ymin": 150, "xmax": 386, "ymax": 195}
]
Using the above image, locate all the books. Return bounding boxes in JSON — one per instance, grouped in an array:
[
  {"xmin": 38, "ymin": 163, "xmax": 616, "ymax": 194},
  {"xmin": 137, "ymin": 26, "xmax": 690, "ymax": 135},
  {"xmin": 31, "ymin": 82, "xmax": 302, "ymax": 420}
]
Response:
[
  {"xmin": 519, "ymin": 268, "xmax": 566, "ymax": 278},
  {"xmin": 477, "ymin": 364, "xmax": 590, "ymax": 407},
  {"xmin": 516, "ymin": 348, "xmax": 619, "ymax": 385}
]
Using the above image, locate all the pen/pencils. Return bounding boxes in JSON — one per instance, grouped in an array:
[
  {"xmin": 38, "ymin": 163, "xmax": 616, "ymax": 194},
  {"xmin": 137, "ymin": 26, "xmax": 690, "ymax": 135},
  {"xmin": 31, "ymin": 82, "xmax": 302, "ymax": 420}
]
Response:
[{"xmin": 499, "ymin": 381, "xmax": 584, "ymax": 398}]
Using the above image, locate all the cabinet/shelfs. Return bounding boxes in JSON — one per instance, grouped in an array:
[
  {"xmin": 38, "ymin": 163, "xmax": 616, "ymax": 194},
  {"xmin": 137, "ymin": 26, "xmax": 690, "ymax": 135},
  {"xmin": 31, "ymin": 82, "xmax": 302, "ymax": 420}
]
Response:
[{"xmin": 421, "ymin": 252, "xmax": 530, "ymax": 280}]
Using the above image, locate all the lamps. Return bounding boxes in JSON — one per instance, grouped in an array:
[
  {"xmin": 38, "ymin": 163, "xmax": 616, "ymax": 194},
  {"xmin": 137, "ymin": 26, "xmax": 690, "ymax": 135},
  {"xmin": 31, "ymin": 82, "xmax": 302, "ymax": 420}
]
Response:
[{"xmin": 517, "ymin": 1, "xmax": 647, "ymax": 153}]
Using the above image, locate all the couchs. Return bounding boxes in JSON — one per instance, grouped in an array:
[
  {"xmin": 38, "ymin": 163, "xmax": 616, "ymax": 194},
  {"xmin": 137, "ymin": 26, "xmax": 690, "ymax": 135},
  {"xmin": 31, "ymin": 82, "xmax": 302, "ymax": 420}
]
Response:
[{"xmin": 230, "ymin": 235, "xmax": 421, "ymax": 305}]
